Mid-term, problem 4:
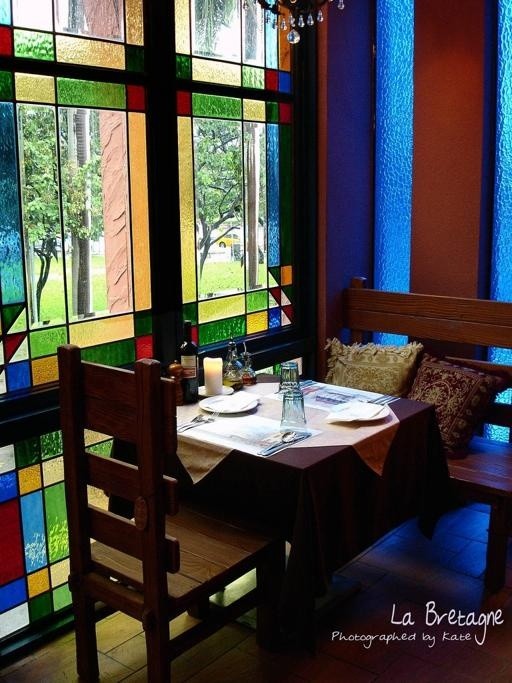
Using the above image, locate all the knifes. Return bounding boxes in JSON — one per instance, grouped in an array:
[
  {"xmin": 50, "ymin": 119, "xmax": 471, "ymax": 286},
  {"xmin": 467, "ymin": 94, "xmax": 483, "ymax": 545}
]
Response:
[{"xmin": 263, "ymin": 433, "xmax": 314, "ymax": 459}]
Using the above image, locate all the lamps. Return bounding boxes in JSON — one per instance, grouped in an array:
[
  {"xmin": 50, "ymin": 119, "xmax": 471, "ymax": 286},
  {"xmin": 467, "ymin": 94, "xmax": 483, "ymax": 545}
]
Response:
[{"xmin": 242, "ymin": 0, "xmax": 346, "ymax": 45}]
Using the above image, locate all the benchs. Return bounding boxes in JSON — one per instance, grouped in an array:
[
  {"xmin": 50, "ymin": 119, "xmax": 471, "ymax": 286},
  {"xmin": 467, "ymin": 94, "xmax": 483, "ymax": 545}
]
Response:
[{"xmin": 325, "ymin": 275, "xmax": 512, "ymax": 596}]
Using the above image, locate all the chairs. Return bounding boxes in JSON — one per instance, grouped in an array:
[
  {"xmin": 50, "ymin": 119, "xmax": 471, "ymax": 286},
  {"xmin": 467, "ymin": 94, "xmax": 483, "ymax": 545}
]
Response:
[{"xmin": 54, "ymin": 343, "xmax": 285, "ymax": 683}]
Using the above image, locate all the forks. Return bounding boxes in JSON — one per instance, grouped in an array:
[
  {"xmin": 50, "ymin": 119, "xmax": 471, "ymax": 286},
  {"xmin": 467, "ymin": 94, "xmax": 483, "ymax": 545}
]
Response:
[{"xmin": 177, "ymin": 408, "xmax": 221, "ymax": 433}]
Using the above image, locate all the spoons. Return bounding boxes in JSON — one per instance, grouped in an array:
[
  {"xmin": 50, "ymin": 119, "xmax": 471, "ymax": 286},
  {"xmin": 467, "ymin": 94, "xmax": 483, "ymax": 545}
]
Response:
[{"xmin": 258, "ymin": 431, "xmax": 296, "ymax": 455}]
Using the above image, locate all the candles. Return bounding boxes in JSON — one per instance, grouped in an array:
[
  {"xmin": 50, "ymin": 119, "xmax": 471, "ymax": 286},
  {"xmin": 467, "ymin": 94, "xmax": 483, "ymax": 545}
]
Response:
[{"xmin": 203, "ymin": 356, "xmax": 224, "ymax": 394}]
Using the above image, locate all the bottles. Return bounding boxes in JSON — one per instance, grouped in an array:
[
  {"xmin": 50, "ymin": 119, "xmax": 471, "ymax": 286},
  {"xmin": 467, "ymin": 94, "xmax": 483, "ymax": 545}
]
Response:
[
  {"xmin": 179, "ymin": 320, "xmax": 199, "ymax": 404},
  {"xmin": 221, "ymin": 332, "xmax": 257, "ymax": 394}
]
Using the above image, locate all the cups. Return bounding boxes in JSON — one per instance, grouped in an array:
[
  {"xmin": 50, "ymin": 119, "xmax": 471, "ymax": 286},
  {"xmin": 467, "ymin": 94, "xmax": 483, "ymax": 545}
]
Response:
[{"xmin": 279, "ymin": 362, "xmax": 309, "ymax": 440}]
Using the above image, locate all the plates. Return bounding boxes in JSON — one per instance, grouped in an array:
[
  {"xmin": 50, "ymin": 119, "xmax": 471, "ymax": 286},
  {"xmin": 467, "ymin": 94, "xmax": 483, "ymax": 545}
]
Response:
[
  {"xmin": 357, "ymin": 405, "xmax": 391, "ymax": 423},
  {"xmin": 197, "ymin": 386, "xmax": 258, "ymax": 415}
]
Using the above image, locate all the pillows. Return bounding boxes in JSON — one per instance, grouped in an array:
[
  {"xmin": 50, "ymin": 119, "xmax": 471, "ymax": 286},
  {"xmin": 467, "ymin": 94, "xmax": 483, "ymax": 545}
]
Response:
[
  {"xmin": 323, "ymin": 336, "xmax": 425, "ymax": 397},
  {"xmin": 407, "ymin": 352, "xmax": 512, "ymax": 460}
]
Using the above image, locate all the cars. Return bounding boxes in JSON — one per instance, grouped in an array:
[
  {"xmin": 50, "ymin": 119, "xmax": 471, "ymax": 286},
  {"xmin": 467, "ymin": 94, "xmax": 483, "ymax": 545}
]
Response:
[{"xmin": 216, "ymin": 233, "xmax": 241, "ymax": 248}]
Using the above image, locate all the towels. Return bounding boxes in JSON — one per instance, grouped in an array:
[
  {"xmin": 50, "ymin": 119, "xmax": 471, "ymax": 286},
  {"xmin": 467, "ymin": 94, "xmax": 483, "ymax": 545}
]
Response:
[
  {"xmin": 326, "ymin": 400, "xmax": 386, "ymax": 424},
  {"xmin": 207, "ymin": 390, "xmax": 262, "ymax": 411}
]
